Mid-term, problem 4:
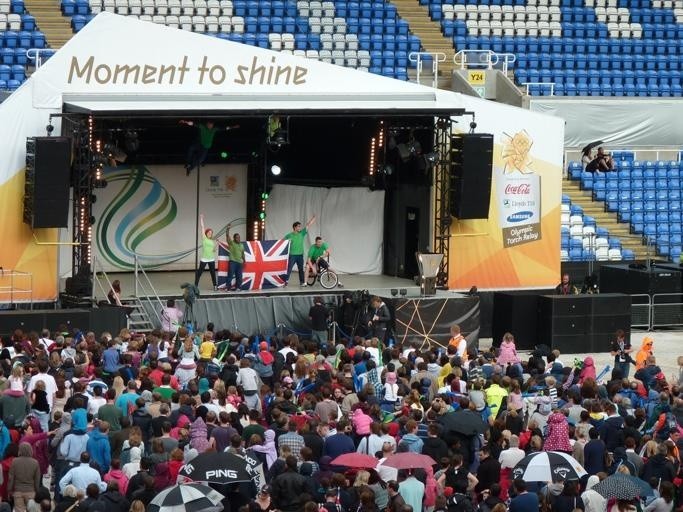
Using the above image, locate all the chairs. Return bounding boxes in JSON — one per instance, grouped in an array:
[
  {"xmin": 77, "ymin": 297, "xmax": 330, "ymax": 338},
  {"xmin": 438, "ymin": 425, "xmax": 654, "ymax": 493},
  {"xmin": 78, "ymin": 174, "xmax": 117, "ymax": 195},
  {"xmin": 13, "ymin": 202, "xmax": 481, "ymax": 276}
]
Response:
[
  {"xmin": 0, "ymin": 0, "xmax": 54, "ymax": 91},
  {"xmin": 421, "ymin": 0, "xmax": 683, "ymax": 97},
  {"xmin": 552, "ymin": 153, "xmax": 682, "ymax": 266},
  {"xmin": 61, "ymin": 2, "xmax": 435, "ymax": 81}
]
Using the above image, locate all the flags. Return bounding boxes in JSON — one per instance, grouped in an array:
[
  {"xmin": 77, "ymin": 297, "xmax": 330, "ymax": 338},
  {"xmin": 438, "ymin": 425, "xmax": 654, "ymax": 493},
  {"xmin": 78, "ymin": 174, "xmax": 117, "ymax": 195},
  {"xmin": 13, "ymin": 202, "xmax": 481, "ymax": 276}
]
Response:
[{"xmin": 217, "ymin": 238, "xmax": 291, "ymax": 291}]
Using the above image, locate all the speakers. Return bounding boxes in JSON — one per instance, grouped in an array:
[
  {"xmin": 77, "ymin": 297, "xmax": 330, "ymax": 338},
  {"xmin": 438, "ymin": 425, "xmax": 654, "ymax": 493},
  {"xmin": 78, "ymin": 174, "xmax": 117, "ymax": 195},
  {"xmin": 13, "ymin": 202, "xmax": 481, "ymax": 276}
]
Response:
[
  {"xmin": 449, "ymin": 133, "xmax": 494, "ymax": 219},
  {"xmin": 22, "ymin": 134, "xmax": 72, "ymax": 228}
]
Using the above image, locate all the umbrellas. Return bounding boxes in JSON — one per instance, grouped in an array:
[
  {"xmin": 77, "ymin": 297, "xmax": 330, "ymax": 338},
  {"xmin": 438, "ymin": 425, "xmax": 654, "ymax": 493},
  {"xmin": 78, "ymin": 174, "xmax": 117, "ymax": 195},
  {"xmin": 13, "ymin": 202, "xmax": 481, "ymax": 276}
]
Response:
[{"xmin": 581, "ymin": 141, "xmax": 603, "ymax": 152}]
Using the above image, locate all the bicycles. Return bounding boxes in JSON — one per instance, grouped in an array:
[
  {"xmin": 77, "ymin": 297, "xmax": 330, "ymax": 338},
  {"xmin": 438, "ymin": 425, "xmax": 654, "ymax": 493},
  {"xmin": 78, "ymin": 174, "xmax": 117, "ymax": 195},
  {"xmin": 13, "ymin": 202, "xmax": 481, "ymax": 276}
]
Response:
[{"xmin": 307, "ymin": 259, "xmax": 338, "ymax": 289}]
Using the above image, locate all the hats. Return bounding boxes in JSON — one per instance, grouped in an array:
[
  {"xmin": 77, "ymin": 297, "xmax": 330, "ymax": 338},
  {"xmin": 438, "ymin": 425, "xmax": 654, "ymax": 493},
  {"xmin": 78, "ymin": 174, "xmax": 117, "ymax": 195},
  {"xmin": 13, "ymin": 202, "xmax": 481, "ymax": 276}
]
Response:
[
  {"xmin": 283, "ymin": 376, "xmax": 295, "ymax": 384},
  {"xmin": 260, "ymin": 341, "xmax": 268, "ymax": 350},
  {"xmin": 385, "ymin": 372, "xmax": 398, "ymax": 383}
]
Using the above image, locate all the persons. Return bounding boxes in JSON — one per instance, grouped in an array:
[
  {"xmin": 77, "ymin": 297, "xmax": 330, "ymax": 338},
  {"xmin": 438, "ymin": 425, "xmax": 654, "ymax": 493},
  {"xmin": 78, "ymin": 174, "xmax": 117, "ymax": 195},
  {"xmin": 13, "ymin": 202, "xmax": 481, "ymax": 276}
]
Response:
[
  {"xmin": 596, "ymin": 147, "xmax": 617, "ymax": 172},
  {"xmin": 281, "ymin": 215, "xmax": 316, "ymax": 288},
  {"xmin": 195, "ymin": 212, "xmax": 219, "ymax": 291},
  {"xmin": 178, "ymin": 118, "xmax": 241, "ymax": 177},
  {"xmin": 300, "ymin": 236, "xmax": 343, "ymax": 288},
  {"xmin": 224, "ymin": 223, "xmax": 246, "ymax": 293},
  {"xmin": 266, "ymin": 117, "xmax": 283, "ymax": 138},
  {"xmin": 581, "ymin": 149, "xmax": 613, "ymax": 172}
]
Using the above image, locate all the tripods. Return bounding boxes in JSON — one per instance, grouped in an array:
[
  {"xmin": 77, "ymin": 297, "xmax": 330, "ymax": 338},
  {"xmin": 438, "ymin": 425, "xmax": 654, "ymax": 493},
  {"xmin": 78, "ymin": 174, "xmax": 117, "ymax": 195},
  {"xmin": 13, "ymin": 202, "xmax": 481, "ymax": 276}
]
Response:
[
  {"xmin": 350, "ymin": 307, "xmax": 371, "ymax": 341},
  {"xmin": 182, "ymin": 305, "xmax": 195, "ymax": 326}
]
